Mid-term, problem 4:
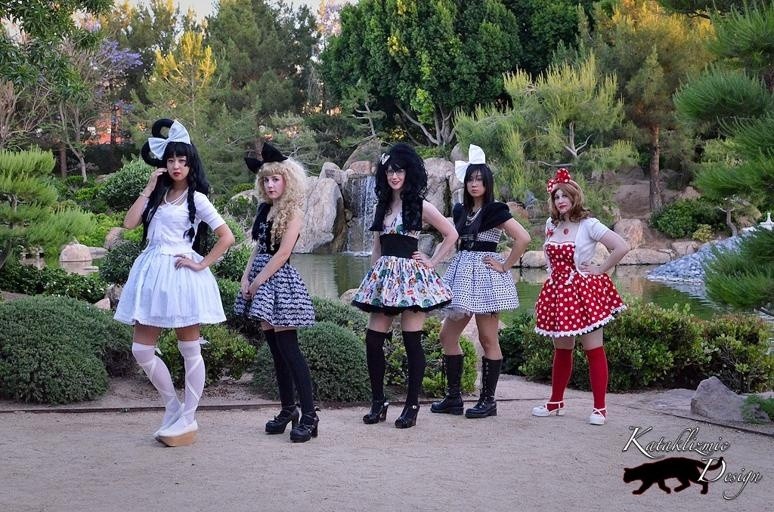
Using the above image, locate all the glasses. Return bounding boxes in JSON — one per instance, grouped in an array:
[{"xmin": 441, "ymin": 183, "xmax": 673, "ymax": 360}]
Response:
[{"xmin": 385, "ymin": 169, "xmax": 405, "ymax": 176}]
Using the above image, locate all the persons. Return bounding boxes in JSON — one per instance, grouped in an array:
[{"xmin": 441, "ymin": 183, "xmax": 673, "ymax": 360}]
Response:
[
  {"xmin": 352, "ymin": 144, "xmax": 459, "ymax": 429},
  {"xmin": 532, "ymin": 168, "xmax": 630, "ymax": 425},
  {"xmin": 430, "ymin": 144, "xmax": 530, "ymax": 418},
  {"xmin": 234, "ymin": 142, "xmax": 319, "ymax": 442},
  {"xmin": 112, "ymin": 118, "xmax": 236, "ymax": 448}
]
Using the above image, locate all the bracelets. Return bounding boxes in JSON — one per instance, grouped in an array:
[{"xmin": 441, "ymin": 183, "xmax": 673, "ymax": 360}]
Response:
[{"xmin": 139, "ymin": 192, "xmax": 152, "ymax": 199}]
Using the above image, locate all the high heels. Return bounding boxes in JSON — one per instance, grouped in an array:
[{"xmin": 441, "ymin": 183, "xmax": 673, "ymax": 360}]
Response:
[
  {"xmin": 363, "ymin": 398, "xmax": 389, "ymax": 424},
  {"xmin": 266, "ymin": 405, "xmax": 299, "ymax": 433},
  {"xmin": 159, "ymin": 416, "xmax": 198, "ymax": 447},
  {"xmin": 290, "ymin": 409, "xmax": 319, "ymax": 442},
  {"xmin": 153, "ymin": 402, "xmax": 185, "ymax": 442},
  {"xmin": 396, "ymin": 404, "xmax": 419, "ymax": 428},
  {"xmin": 532, "ymin": 401, "xmax": 565, "ymax": 417},
  {"xmin": 589, "ymin": 408, "xmax": 608, "ymax": 425}
]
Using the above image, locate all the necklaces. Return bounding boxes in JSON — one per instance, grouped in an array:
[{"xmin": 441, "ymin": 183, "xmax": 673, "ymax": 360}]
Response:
[
  {"xmin": 164, "ymin": 185, "xmax": 183, "ymax": 204},
  {"xmin": 561, "ymin": 221, "xmax": 574, "ymax": 235},
  {"xmin": 465, "ymin": 207, "xmax": 482, "ymax": 226}
]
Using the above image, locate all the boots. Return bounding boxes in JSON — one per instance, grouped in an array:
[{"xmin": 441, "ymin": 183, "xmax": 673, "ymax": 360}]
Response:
[
  {"xmin": 431, "ymin": 355, "xmax": 464, "ymax": 415},
  {"xmin": 466, "ymin": 357, "xmax": 502, "ymax": 418}
]
